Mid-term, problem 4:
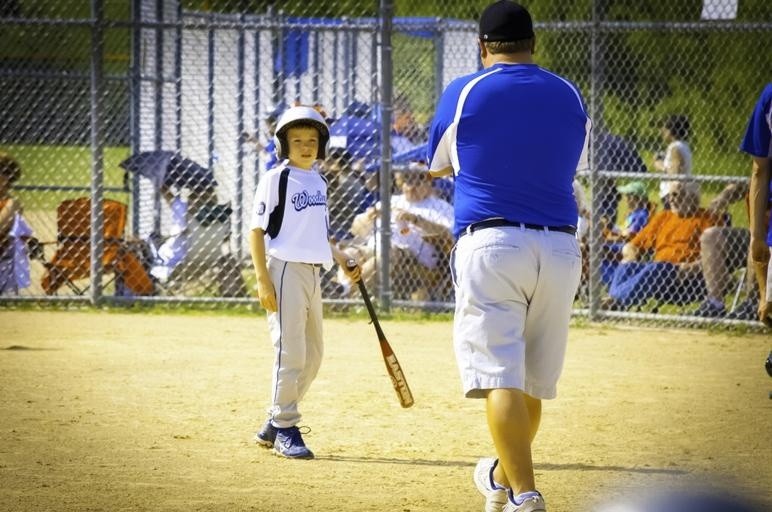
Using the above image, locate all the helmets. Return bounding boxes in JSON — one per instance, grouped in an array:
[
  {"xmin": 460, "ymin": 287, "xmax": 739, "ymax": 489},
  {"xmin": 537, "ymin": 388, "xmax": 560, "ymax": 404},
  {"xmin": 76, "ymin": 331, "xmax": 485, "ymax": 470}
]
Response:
[{"xmin": 274, "ymin": 107, "xmax": 330, "ymax": 160}]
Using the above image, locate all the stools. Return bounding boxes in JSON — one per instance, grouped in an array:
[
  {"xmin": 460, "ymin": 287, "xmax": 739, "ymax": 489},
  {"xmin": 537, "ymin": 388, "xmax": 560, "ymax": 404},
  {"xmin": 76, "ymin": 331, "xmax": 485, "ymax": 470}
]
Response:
[{"xmin": 729, "ymin": 256, "xmax": 752, "ymax": 313}]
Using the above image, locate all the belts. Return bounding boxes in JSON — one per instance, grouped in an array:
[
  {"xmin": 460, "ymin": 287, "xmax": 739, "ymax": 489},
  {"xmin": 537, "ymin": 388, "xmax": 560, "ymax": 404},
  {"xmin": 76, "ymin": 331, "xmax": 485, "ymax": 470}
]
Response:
[{"xmin": 461, "ymin": 218, "xmax": 576, "ymax": 235}]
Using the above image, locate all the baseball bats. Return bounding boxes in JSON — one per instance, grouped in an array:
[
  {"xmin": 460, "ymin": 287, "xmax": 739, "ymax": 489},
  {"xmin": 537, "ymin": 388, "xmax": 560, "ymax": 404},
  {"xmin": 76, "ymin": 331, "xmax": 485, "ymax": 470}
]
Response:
[{"xmin": 347, "ymin": 259, "xmax": 413, "ymax": 407}]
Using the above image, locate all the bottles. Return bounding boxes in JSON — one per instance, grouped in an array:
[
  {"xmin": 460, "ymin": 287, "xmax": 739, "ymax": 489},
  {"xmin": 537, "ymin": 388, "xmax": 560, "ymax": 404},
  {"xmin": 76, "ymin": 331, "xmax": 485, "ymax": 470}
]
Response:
[{"xmin": 395, "ymin": 214, "xmax": 410, "ymax": 235}]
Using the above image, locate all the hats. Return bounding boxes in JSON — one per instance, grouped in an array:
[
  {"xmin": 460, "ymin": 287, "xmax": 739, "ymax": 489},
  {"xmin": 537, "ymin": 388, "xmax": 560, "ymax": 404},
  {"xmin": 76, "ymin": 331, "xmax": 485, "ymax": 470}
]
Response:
[
  {"xmin": 617, "ymin": 183, "xmax": 647, "ymax": 204},
  {"xmin": 479, "ymin": 1, "xmax": 533, "ymax": 41}
]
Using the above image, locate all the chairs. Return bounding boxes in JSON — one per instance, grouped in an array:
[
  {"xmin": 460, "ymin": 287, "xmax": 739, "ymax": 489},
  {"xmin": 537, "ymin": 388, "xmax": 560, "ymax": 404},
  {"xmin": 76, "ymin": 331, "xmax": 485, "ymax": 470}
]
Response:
[
  {"xmin": 28, "ymin": 196, "xmax": 151, "ymax": 297},
  {"xmin": 154, "ymin": 201, "xmax": 233, "ymax": 299},
  {"xmin": 600, "ymin": 201, "xmax": 657, "ymax": 244},
  {"xmin": 632, "ymin": 211, "xmax": 731, "ymax": 313},
  {"xmin": 0, "ymin": 211, "xmax": 30, "ymax": 298}
]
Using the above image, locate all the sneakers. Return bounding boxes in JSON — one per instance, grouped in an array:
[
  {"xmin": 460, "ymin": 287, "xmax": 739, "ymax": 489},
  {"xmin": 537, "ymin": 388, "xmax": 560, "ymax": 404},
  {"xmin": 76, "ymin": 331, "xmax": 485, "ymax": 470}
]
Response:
[
  {"xmin": 474, "ymin": 458, "xmax": 546, "ymax": 512},
  {"xmin": 256, "ymin": 420, "xmax": 313, "ymax": 459}
]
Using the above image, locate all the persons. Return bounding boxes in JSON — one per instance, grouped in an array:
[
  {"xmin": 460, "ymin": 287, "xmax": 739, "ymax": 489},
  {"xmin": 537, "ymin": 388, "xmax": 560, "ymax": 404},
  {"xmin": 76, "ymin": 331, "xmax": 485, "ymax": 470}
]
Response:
[
  {"xmin": 572, "ymin": 115, "xmax": 772, "ymax": 324},
  {"xmin": 739, "ymin": 78, "xmax": 772, "ymax": 414},
  {"xmin": 425, "ymin": 0, "xmax": 588, "ymax": 512},
  {"xmin": 1, "ymin": 92, "xmax": 454, "ymax": 303},
  {"xmin": 248, "ymin": 104, "xmax": 363, "ymax": 464}
]
